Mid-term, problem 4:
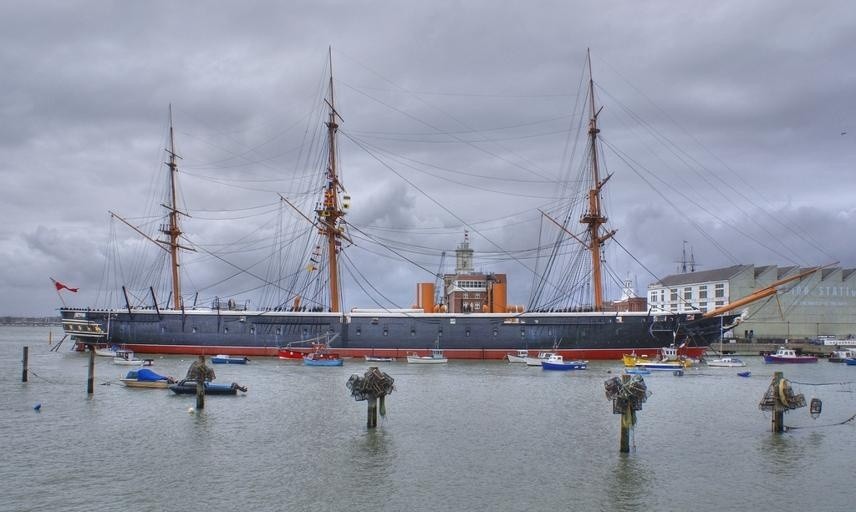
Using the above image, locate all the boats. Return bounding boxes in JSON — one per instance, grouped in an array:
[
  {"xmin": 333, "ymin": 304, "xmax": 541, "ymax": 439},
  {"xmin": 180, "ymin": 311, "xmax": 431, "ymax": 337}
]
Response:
[
  {"xmin": 92, "ymin": 345, "xmax": 250, "ymax": 394},
  {"xmin": 277, "ymin": 347, "xmax": 449, "ymax": 366},
  {"xmin": 829, "ymin": 351, "xmax": 856, "ymax": 365},
  {"xmin": 621, "ymin": 347, "xmax": 751, "ymax": 377},
  {"xmin": 763, "ymin": 347, "xmax": 817, "ymax": 363},
  {"xmin": 507, "ymin": 352, "xmax": 587, "ymax": 371}
]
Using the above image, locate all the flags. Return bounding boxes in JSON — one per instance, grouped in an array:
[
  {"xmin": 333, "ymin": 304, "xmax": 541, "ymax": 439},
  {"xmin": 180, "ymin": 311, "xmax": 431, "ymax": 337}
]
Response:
[{"xmin": 49, "ymin": 277, "xmax": 80, "ymax": 293}]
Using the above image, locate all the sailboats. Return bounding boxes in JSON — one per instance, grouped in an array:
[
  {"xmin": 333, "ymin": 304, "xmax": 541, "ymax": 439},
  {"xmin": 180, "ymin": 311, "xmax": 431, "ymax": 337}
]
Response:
[{"xmin": 53, "ymin": 46, "xmax": 843, "ymax": 361}]
